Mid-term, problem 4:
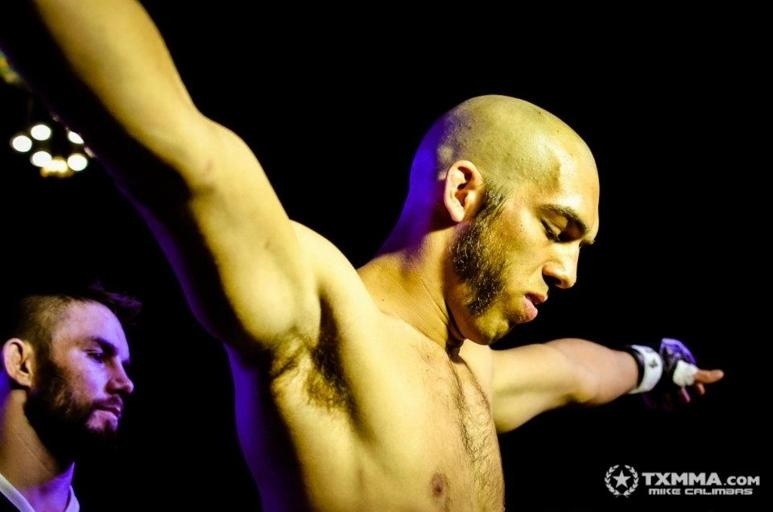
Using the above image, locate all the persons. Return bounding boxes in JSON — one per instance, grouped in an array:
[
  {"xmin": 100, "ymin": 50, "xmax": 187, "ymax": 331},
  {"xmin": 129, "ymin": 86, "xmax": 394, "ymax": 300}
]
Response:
[
  {"xmin": 0, "ymin": 0, "xmax": 724, "ymax": 512},
  {"xmin": 0, "ymin": 279, "xmax": 135, "ymax": 511}
]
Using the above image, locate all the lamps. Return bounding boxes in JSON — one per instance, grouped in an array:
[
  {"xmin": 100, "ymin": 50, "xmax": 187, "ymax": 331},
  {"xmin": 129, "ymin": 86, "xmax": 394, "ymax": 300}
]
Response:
[{"xmin": 12, "ymin": 119, "xmax": 99, "ymax": 182}]
